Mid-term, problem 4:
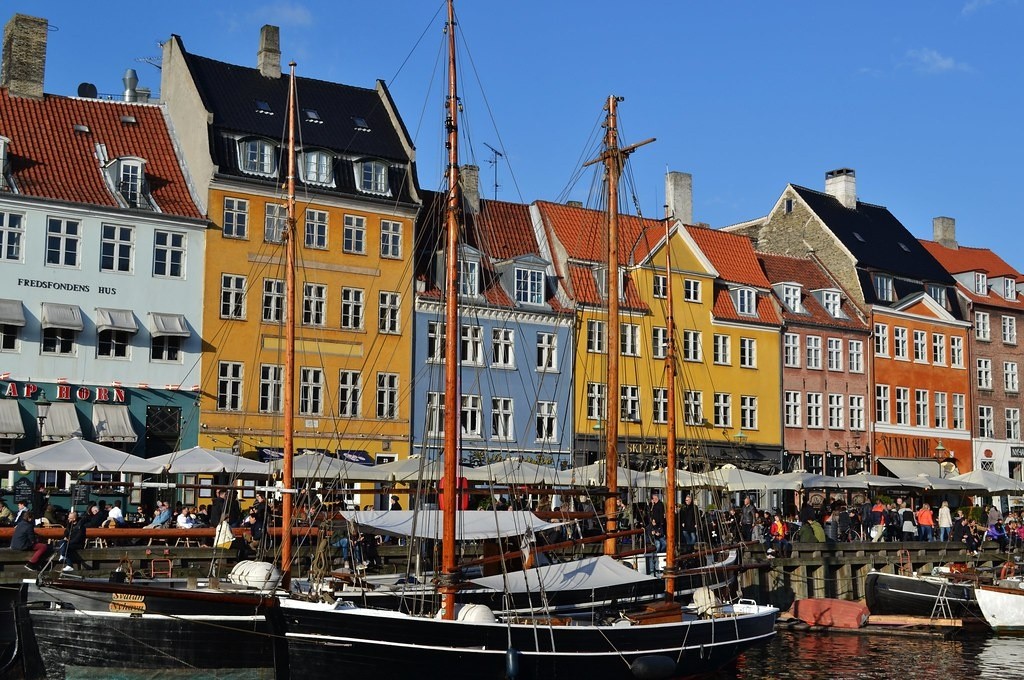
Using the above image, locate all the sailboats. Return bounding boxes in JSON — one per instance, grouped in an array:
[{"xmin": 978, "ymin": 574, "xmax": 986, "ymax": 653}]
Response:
[{"xmin": 18, "ymin": 1, "xmax": 791, "ymax": 680}]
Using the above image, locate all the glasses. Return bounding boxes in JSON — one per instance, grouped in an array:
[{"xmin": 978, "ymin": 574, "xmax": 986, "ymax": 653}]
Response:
[{"xmin": 137, "ymin": 508, "xmax": 142, "ymax": 511}]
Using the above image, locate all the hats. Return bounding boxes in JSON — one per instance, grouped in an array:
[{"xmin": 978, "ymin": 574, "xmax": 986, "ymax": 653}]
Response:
[{"xmin": 391, "ymin": 495, "xmax": 399, "ymax": 501}]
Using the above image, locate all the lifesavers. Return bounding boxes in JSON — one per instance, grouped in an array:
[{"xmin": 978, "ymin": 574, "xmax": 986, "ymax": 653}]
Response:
[{"xmin": 1001, "ymin": 562, "xmax": 1016, "ymax": 580}]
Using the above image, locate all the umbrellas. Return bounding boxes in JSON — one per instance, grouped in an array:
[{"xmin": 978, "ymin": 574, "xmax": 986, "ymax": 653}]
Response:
[{"xmin": 0, "ymin": 436, "xmax": 1024, "ymax": 493}]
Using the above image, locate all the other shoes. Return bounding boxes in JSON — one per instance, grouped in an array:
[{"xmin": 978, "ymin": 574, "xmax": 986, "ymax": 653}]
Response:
[
  {"xmin": 62, "ymin": 565, "xmax": 73, "ymax": 571},
  {"xmin": 201, "ymin": 543, "xmax": 211, "ymax": 548},
  {"xmin": 59, "ymin": 555, "xmax": 66, "ymax": 561},
  {"xmin": 24, "ymin": 561, "xmax": 38, "ymax": 572}
]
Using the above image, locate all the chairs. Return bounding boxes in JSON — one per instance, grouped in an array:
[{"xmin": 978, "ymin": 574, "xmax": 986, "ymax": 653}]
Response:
[{"xmin": 39, "ymin": 517, "xmax": 202, "ymax": 548}]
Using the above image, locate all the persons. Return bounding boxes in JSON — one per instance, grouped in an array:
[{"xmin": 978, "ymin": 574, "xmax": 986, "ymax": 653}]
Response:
[{"xmin": 7, "ymin": 491, "xmax": 1024, "ymax": 562}]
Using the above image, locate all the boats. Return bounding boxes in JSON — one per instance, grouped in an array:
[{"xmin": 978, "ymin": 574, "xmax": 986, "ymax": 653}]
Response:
[
  {"xmin": 864, "ymin": 567, "xmax": 986, "ymax": 624},
  {"xmin": 972, "ymin": 576, "xmax": 1024, "ymax": 634},
  {"xmin": 787, "ymin": 596, "xmax": 871, "ymax": 629}
]
